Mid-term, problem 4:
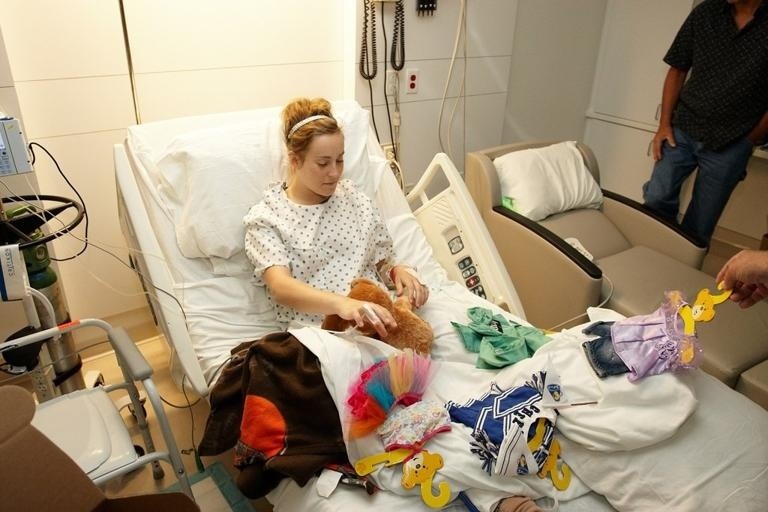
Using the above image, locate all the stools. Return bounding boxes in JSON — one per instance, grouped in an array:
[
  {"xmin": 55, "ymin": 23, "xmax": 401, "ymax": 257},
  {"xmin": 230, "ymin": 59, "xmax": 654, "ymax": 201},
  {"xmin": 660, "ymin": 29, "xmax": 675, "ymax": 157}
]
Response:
[{"xmin": 600, "ymin": 238, "xmax": 767, "ymax": 391}]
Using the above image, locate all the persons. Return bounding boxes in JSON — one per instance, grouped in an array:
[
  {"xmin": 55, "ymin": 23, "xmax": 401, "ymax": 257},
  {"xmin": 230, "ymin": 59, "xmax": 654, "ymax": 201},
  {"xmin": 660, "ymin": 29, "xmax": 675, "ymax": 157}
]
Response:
[
  {"xmin": 713, "ymin": 247, "xmax": 768, "ymax": 307},
  {"xmin": 642, "ymin": 0, "xmax": 767, "ymax": 253},
  {"xmin": 245, "ymin": 95, "xmax": 432, "ymax": 339}
]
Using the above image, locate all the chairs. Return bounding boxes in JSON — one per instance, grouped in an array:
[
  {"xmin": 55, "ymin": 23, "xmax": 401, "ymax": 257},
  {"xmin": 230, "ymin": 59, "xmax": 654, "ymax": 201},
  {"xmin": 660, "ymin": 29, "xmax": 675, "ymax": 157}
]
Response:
[
  {"xmin": 459, "ymin": 137, "xmax": 710, "ymax": 334},
  {"xmin": 0, "ymin": 316, "xmax": 201, "ymax": 512}
]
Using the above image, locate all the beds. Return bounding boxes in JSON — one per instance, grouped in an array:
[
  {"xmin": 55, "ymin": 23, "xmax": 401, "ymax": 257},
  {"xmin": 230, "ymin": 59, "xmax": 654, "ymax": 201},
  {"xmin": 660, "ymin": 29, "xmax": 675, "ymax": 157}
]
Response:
[{"xmin": 111, "ymin": 101, "xmax": 614, "ymax": 511}]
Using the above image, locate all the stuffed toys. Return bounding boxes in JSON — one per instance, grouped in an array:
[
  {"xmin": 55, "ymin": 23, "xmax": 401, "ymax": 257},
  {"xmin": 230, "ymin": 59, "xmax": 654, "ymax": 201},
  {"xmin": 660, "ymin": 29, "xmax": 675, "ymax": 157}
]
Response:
[{"xmin": 325, "ymin": 277, "xmax": 433, "ymax": 351}]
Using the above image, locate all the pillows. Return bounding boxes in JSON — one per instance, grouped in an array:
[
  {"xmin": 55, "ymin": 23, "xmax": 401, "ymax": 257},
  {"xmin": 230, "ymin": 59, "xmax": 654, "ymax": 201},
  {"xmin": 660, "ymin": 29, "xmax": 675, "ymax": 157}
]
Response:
[
  {"xmin": 489, "ymin": 139, "xmax": 606, "ymax": 223},
  {"xmin": 493, "ymin": 319, "xmax": 768, "ymax": 511},
  {"xmin": 124, "ymin": 106, "xmax": 372, "ymax": 269}
]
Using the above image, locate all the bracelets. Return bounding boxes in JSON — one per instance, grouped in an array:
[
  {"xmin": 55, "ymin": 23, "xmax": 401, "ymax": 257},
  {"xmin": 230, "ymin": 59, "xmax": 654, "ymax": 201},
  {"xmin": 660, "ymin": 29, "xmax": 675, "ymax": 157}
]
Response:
[{"xmin": 386, "ymin": 265, "xmax": 396, "ymax": 284}]
